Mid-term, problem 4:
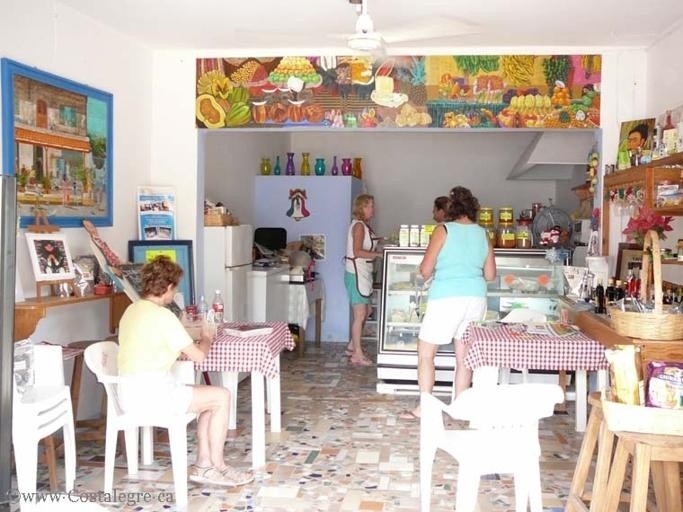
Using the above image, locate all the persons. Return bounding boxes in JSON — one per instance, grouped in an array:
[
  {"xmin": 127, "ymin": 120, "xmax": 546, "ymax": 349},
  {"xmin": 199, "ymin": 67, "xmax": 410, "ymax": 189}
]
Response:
[
  {"xmin": 159, "ymin": 226, "xmax": 170, "ymax": 240},
  {"xmin": 115, "ymin": 255, "xmax": 257, "ymax": 487},
  {"xmin": 40, "ymin": 239, "xmax": 66, "ymax": 274},
  {"xmin": 343, "ymin": 194, "xmax": 384, "ymax": 367},
  {"xmin": 432, "ymin": 195, "xmax": 450, "ymax": 222},
  {"xmin": 411, "ymin": 185, "xmax": 497, "ymax": 417},
  {"xmin": 626, "ymin": 123, "xmax": 649, "ymax": 151},
  {"xmin": 34, "ymin": 174, "xmax": 104, "ymax": 214},
  {"xmin": 145, "ymin": 227, "xmax": 156, "ymax": 240}
]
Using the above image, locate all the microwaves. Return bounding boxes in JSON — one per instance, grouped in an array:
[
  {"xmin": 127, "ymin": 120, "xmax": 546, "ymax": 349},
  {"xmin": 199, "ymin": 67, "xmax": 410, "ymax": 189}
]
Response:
[{"xmin": 574, "ymin": 219, "xmax": 590, "ymax": 244}]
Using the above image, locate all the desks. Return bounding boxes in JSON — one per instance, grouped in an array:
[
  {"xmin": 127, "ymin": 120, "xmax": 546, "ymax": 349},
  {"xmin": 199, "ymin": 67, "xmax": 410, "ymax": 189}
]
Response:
[
  {"xmin": 560, "ymin": 293, "xmax": 683, "ymax": 376},
  {"xmin": 286, "ymin": 277, "xmax": 328, "ymax": 359}
]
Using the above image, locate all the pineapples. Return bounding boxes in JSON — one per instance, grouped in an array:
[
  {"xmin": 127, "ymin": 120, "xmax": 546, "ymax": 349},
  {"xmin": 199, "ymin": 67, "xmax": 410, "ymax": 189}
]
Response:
[{"xmin": 396, "ymin": 59, "xmax": 428, "ymax": 105}]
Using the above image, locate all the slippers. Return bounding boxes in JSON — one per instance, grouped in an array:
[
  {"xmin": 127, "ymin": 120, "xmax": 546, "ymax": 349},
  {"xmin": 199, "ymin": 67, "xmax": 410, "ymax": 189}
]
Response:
[
  {"xmin": 350, "ymin": 357, "xmax": 372, "ymax": 365},
  {"xmin": 344, "ymin": 346, "xmax": 368, "ymax": 356},
  {"xmin": 396, "ymin": 410, "xmax": 420, "ymax": 422}
]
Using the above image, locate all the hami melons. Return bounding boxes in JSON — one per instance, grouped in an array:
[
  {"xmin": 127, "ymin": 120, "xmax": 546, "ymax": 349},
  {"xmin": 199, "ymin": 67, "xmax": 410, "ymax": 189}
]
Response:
[{"xmin": 196, "ymin": 85, "xmax": 251, "ymax": 128}]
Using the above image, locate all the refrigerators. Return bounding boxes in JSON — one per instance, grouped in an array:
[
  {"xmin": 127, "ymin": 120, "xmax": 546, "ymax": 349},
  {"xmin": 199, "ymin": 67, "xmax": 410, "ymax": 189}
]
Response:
[{"xmin": 203, "ymin": 225, "xmax": 253, "ymax": 384}]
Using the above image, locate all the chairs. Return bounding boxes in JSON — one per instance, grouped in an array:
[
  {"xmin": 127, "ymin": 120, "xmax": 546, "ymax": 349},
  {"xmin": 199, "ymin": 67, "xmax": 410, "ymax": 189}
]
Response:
[
  {"xmin": 419, "ymin": 380, "xmax": 563, "ymax": 511},
  {"xmin": 255, "ymin": 226, "xmax": 288, "ymax": 260}
]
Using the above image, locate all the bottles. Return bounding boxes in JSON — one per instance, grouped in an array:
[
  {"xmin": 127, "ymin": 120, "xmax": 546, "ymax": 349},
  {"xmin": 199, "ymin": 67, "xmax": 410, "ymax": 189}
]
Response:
[
  {"xmin": 399, "ymin": 224, "xmax": 409, "ymax": 247},
  {"xmin": 197, "ymin": 295, "xmax": 208, "ymax": 321},
  {"xmin": 301, "ymin": 152, "xmax": 310, "ymax": 175},
  {"xmin": 314, "ymin": 158, "xmax": 325, "ymax": 175},
  {"xmin": 409, "ymin": 225, "xmax": 420, "ymax": 247},
  {"xmin": 664, "ymin": 287, "xmax": 674, "ymax": 304},
  {"xmin": 625, "ymin": 261, "xmax": 635, "ymax": 297},
  {"xmin": 332, "ymin": 156, "xmax": 338, "ymax": 174},
  {"xmin": 342, "ymin": 158, "xmax": 352, "ymax": 175},
  {"xmin": 353, "ymin": 158, "xmax": 362, "ymax": 178},
  {"xmin": 419, "ymin": 224, "xmax": 431, "ymax": 246},
  {"xmin": 594, "ymin": 277, "xmax": 604, "ymax": 313},
  {"xmin": 615, "ymin": 280, "xmax": 625, "ymax": 300},
  {"xmin": 212, "ymin": 289, "xmax": 224, "ymax": 325},
  {"xmin": 273, "ymin": 156, "xmax": 281, "ymax": 174},
  {"xmin": 285, "ymin": 152, "xmax": 296, "ymax": 175},
  {"xmin": 636, "ymin": 267, "xmax": 642, "ymax": 299},
  {"xmin": 605, "ymin": 277, "xmax": 615, "ymax": 314},
  {"xmin": 675, "ymin": 286, "xmax": 683, "ymax": 303},
  {"xmin": 260, "ymin": 157, "xmax": 270, "ymax": 174}
]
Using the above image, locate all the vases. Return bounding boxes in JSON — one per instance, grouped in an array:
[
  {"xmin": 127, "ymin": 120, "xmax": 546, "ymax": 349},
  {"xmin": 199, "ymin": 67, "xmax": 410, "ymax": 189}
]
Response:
[{"xmin": 259, "ymin": 151, "xmax": 363, "ymax": 180}]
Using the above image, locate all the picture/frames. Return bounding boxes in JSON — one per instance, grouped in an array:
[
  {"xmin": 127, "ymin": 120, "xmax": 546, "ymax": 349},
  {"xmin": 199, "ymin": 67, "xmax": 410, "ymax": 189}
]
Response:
[
  {"xmin": 126, "ymin": 239, "xmax": 196, "ymax": 307},
  {"xmin": 1, "ymin": 58, "xmax": 117, "ymax": 229},
  {"xmin": 23, "ymin": 231, "xmax": 78, "ymax": 284},
  {"xmin": 614, "ymin": 241, "xmax": 655, "ymax": 286}
]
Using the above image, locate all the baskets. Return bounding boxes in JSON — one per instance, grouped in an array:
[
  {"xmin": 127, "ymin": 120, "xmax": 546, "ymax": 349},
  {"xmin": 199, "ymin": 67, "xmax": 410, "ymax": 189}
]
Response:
[
  {"xmin": 605, "ymin": 229, "xmax": 683, "ymax": 340},
  {"xmin": 204, "ymin": 213, "xmax": 233, "ymax": 226}
]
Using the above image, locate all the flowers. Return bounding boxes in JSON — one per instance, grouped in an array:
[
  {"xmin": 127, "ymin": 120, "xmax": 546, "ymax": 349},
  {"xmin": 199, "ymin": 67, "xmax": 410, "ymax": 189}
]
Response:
[{"xmin": 623, "ymin": 207, "xmax": 677, "ymax": 245}]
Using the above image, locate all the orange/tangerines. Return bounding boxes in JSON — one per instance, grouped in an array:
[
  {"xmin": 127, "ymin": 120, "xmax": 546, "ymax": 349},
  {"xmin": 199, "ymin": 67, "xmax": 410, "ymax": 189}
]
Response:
[{"xmin": 550, "ymin": 83, "xmax": 572, "ymax": 106}]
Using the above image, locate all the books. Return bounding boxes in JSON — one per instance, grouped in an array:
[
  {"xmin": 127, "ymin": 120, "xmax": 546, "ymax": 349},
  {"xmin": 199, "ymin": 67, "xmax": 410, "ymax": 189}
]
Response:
[
  {"xmin": 223, "ymin": 323, "xmax": 274, "ymax": 337},
  {"xmin": 526, "ymin": 320, "xmax": 580, "ymax": 338}
]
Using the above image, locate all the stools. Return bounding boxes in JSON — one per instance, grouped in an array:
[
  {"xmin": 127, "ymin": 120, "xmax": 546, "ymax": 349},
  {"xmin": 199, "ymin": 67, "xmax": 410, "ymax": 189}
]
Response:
[{"xmin": 563, "ymin": 389, "xmax": 683, "ymax": 512}]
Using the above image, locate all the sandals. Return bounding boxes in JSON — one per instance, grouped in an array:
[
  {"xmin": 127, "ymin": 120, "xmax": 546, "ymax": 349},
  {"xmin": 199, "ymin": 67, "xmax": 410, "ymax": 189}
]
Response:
[
  {"xmin": 221, "ymin": 464, "xmax": 255, "ymax": 486},
  {"xmin": 188, "ymin": 465, "xmax": 237, "ymax": 488}
]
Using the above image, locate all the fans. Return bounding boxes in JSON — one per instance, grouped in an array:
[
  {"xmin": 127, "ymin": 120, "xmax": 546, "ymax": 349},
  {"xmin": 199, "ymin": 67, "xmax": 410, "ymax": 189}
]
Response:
[
  {"xmin": 326, "ymin": 1, "xmax": 471, "ymax": 53},
  {"xmin": 530, "ymin": 204, "xmax": 574, "ymax": 249}
]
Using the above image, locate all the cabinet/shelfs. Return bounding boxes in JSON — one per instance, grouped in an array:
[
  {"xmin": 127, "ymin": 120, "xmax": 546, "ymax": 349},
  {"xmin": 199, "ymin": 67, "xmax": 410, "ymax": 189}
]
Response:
[
  {"xmin": 373, "ymin": 246, "xmax": 574, "ymax": 387},
  {"xmin": 601, "ymin": 150, "xmax": 683, "ymax": 268},
  {"xmin": 359, "ymin": 284, "xmax": 382, "ymax": 341}
]
